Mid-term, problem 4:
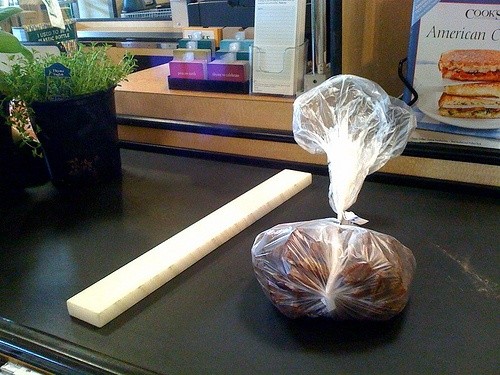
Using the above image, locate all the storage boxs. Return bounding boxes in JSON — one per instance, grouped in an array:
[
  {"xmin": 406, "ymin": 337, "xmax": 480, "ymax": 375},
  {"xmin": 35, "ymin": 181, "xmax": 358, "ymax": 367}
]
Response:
[{"xmin": 0, "ymin": 0, "xmax": 79, "ymax": 52}]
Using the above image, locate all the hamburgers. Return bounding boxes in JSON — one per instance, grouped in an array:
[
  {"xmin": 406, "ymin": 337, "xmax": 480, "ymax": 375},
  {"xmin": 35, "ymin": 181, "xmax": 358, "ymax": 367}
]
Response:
[{"xmin": 438, "ymin": 49, "xmax": 500, "ymax": 120}]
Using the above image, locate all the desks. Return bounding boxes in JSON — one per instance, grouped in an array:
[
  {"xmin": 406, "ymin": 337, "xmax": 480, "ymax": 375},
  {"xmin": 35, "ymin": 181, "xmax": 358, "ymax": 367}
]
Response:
[{"xmin": 0, "ymin": 130, "xmax": 500, "ymax": 375}]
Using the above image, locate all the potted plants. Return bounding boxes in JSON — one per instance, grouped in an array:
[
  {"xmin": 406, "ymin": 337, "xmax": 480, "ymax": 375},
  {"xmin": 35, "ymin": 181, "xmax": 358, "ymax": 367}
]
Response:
[{"xmin": 0, "ymin": 5, "xmax": 139, "ymax": 200}]
